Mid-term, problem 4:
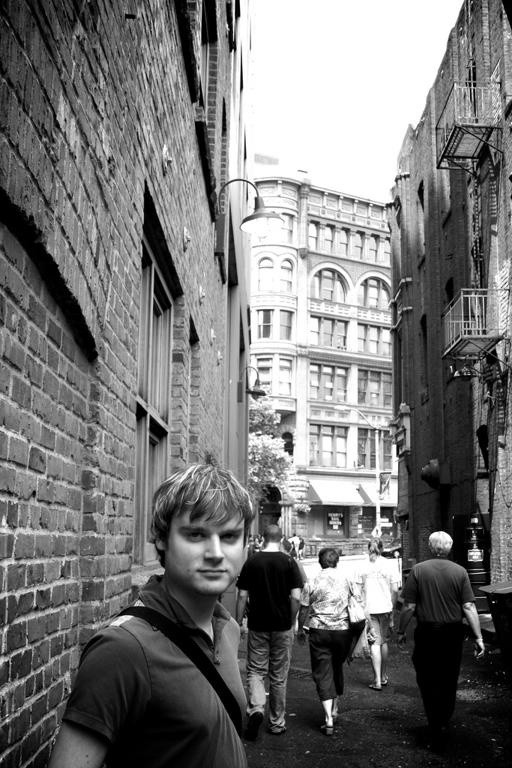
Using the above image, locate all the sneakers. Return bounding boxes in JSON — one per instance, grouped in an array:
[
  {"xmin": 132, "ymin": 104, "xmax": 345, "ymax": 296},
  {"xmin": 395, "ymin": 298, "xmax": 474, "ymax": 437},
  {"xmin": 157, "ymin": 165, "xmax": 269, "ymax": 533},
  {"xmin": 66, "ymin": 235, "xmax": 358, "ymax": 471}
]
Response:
[{"xmin": 242, "ymin": 713, "xmax": 286, "ymax": 741}]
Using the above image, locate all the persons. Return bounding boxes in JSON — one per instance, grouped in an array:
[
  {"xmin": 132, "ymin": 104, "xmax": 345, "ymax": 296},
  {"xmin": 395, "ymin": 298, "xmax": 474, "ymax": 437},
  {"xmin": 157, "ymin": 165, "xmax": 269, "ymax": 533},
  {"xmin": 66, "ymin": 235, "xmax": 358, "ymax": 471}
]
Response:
[
  {"xmin": 394, "ymin": 527, "xmax": 488, "ymax": 744},
  {"xmin": 297, "ymin": 546, "xmax": 364, "ymax": 738},
  {"xmin": 243, "ymin": 530, "xmax": 307, "ymax": 560},
  {"xmin": 357, "ymin": 532, "xmax": 402, "ymax": 697},
  {"xmin": 40, "ymin": 458, "xmax": 256, "ymax": 768},
  {"xmin": 234, "ymin": 522, "xmax": 302, "ymax": 743}
]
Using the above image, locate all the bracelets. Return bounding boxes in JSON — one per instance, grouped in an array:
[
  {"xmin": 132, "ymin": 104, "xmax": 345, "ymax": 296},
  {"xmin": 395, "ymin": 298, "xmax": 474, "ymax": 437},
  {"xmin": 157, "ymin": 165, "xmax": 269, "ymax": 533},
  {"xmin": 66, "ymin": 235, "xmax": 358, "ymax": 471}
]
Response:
[{"xmin": 474, "ymin": 637, "xmax": 483, "ymax": 641}]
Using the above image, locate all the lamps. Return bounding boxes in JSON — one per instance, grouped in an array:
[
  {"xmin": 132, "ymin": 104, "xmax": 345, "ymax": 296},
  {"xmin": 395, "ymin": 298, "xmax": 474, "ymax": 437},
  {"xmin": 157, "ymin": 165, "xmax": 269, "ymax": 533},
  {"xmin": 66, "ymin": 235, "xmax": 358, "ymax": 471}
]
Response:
[
  {"xmin": 453, "ymin": 362, "xmax": 489, "ymax": 381},
  {"xmin": 234, "ymin": 364, "xmax": 267, "ymax": 403},
  {"xmin": 214, "ymin": 179, "xmax": 286, "ymax": 254}
]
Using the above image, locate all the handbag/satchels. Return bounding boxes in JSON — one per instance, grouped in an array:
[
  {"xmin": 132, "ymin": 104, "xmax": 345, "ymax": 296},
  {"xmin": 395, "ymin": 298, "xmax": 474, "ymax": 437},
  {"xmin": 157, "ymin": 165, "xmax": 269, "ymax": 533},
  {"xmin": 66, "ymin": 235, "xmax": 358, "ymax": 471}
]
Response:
[{"xmin": 346, "ymin": 585, "xmax": 365, "ymax": 624}]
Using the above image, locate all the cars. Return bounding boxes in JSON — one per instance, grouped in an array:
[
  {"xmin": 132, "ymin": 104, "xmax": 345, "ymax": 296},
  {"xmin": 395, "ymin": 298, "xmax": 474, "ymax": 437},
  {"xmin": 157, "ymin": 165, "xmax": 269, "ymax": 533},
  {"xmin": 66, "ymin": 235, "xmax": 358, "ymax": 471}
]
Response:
[{"xmin": 381, "ymin": 533, "xmax": 402, "ymax": 557}]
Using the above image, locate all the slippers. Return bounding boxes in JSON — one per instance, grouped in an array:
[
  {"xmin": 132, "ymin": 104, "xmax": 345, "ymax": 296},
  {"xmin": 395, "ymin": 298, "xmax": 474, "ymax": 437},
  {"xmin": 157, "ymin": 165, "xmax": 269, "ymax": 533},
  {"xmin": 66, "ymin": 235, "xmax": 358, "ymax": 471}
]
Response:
[
  {"xmin": 324, "ymin": 712, "xmax": 339, "ymax": 735},
  {"xmin": 369, "ymin": 676, "xmax": 389, "ymax": 690}
]
